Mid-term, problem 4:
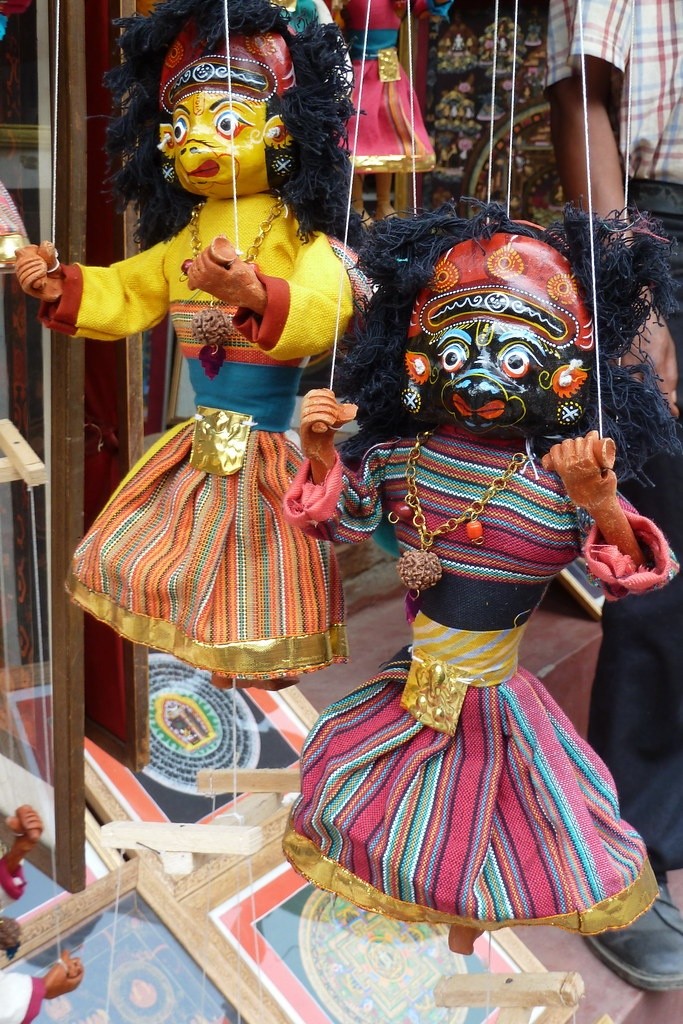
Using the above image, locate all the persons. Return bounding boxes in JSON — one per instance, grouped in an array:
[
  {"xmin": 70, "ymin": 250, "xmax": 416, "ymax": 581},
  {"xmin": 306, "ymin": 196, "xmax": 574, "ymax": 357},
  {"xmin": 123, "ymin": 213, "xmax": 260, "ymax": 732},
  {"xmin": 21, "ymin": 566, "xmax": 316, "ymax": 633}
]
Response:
[
  {"xmin": 12, "ymin": 50, "xmax": 354, "ymax": 682},
  {"xmin": 545, "ymin": 0, "xmax": 683, "ymax": 987},
  {"xmin": 285, "ymin": 201, "xmax": 683, "ymax": 930},
  {"xmin": 328, "ymin": 0, "xmax": 436, "ymax": 226}
]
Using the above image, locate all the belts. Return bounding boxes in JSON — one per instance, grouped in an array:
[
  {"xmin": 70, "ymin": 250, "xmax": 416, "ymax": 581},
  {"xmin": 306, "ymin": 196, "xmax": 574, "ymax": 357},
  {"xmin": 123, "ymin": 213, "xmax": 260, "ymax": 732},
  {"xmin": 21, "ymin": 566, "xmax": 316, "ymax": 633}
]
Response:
[{"xmin": 622, "ymin": 178, "xmax": 683, "ymax": 215}]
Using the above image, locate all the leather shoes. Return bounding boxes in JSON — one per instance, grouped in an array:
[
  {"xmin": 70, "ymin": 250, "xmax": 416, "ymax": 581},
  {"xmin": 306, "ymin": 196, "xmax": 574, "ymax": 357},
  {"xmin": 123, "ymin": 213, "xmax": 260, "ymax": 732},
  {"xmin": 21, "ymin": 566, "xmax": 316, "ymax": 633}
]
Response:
[{"xmin": 585, "ymin": 883, "xmax": 683, "ymax": 991}]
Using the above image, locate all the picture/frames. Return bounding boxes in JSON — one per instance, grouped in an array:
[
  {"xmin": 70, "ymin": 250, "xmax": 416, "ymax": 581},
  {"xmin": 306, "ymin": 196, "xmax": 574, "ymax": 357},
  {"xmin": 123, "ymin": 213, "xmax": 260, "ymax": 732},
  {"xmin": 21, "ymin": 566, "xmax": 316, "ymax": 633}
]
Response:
[{"xmin": 0, "ymin": 648, "xmax": 583, "ymax": 1024}]
